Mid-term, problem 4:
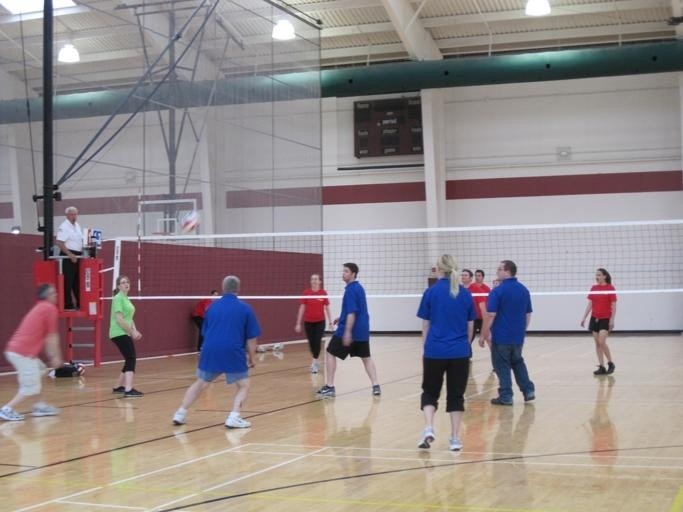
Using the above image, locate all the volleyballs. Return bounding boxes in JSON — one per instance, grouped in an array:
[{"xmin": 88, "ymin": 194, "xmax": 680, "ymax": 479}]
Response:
[{"xmin": 180, "ymin": 211, "xmax": 199, "ymax": 231}]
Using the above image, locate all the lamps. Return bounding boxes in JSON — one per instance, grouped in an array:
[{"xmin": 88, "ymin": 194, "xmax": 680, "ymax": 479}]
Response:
[
  {"xmin": 272, "ymin": 12, "xmax": 296, "ymax": 41},
  {"xmin": 57, "ymin": 31, "xmax": 81, "ymax": 63},
  {"xmin": 526, "ymin": 0, "xmax": 551, "ymax": 17},
  {"xmin": 11, "ymin": 225, "xmax": 21, "ymax": 235}
]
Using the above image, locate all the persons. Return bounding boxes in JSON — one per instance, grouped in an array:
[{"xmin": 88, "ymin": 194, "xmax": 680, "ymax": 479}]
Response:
[
  {"xmin": 417, "ymin": 254, "xmax": 477, "ymax": 453},
  {"xmin": 172, "ymin": 273, "xmax": 261, "ymax": 430},
  {"xmin": 1, "ymin": 280, "xmax": 65, "ymax": 422},
  {"xmin": 578, "ymin": 267, "xmax": 620, "ymax": 377},
  {"xmin": 53, "ymin": 204, "xmax": 87, "ymax": 312},
  {"xmin": 313, "ymin": 264, "xmax": 381, "ymax": 399},
  {"xmin": 107, "ymin": 275, "xmax": 148, "ymax": 397},
  {"xmin": 452, "ymin": 258, "xmax": 537, "ymax": 407},
  {"xmin": 192, "ymin": 289, "xmax": 219, "ymax": 356},
  {"xmin": 294, "ymin": 272, "xmax": 334, "ymax": 373}
]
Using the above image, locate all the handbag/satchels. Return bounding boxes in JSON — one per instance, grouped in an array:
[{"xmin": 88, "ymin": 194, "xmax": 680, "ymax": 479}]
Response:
[{"xmin": 55, "ymin": 363, "xmax": 85, "ymax": 377}]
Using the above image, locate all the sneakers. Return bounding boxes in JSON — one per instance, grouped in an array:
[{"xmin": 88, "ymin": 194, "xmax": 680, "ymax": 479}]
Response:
[
  {"xmin": 124, "ymin": 388, "xmax": 144, "ymax": 397},
  {"xmin": 314, "ymin": 385, "xmax": 335, "ymax": 396},
  {"xmin": 32, "ymin": 404, "xmax": 60, "ymax": 416},
  {"xmin": 492, "ymin": 398, "xmax": 514, "ymax": 404},
  {"xmin": 0, "ymin": 406, "xmax": 24, "ymax": 421},
  {"xmin": 524, "ymin": 392, "xmax": 534, "ymax": 401},
  {"xmin": 224, "ymin": 411, "xmax": 251, "ymax": 428},
  {"xmin": 594, "ymin": 362, "xmax": 615, "ymax": 375},
  {"xmin": 309, "ymin": 364, "xmax": 321, "ymax": 373},
  {"xmin": 418, "ymin": 429, "xmax": 435, "ymax": 448},
  {"xmin": 173, "ymin": 410, "xmax": 187, "ymax": 424},
  {"xmin": 64, "ymin": 306, "xmax": 80, "ymax": 311},
  {"xmin": 113, "ymin": 386, "xmax": 125, "ymax": 392},
  {"xmin": 450, "ymin": 439, "xmax": 462, "ymax": 450},
  {"xmin": 373, "ymin": 385, "xmax": 381, "ymax": 395}
]
